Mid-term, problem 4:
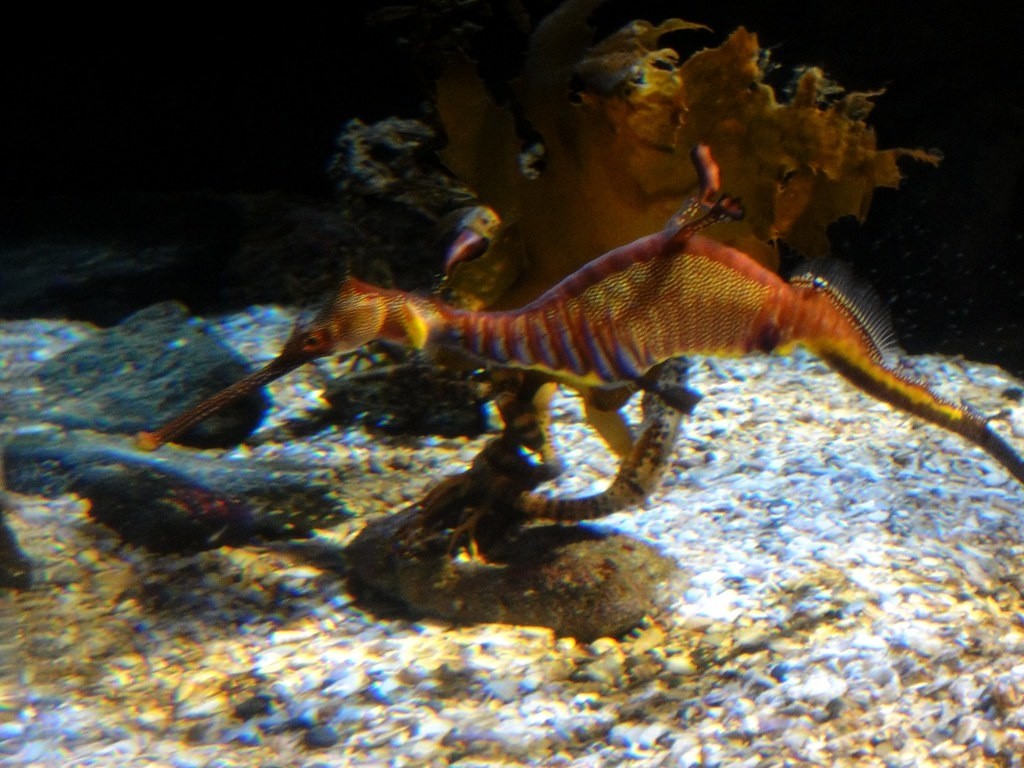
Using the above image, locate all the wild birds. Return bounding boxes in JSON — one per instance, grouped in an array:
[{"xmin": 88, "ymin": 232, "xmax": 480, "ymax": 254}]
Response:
[{"xmin": 429, "ymin": 206, "xmax": 502, "ymax": 294}]
